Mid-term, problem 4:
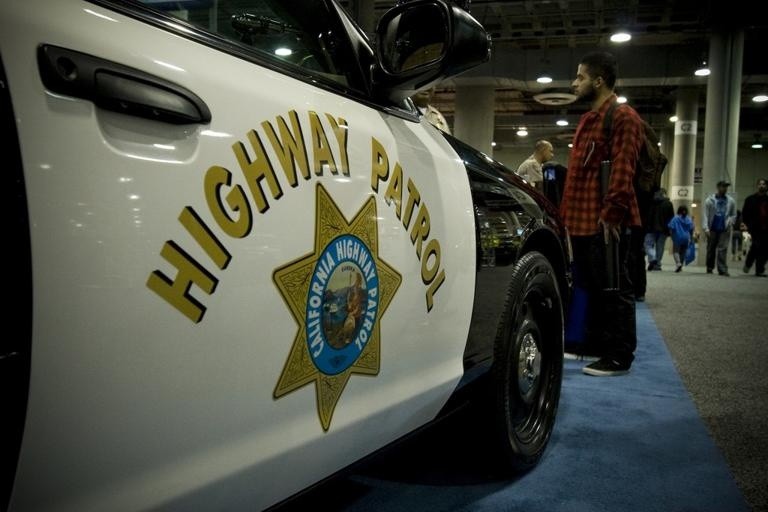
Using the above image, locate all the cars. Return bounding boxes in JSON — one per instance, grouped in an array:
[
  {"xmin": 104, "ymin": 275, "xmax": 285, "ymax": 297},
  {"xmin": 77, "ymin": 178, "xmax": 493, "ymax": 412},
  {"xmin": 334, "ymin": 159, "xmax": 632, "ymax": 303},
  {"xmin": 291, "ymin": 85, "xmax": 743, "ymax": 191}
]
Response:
[{"xmin": 1, "ymin": 0, "xmax": 574, "ymax": 512}]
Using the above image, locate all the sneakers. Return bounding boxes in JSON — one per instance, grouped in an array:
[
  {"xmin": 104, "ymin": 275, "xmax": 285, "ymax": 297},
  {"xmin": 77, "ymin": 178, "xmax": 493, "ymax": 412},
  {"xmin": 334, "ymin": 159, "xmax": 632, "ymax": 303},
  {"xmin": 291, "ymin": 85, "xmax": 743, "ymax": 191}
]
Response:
[{"xmin": 564, "ymin": 342, "xmax": 630, "ymax": 376}]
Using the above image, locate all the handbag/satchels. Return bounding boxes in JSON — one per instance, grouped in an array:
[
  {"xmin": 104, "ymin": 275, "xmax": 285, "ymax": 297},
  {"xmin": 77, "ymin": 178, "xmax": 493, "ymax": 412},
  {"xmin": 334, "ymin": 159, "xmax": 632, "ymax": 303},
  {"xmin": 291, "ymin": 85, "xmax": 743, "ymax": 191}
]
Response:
[{"xmin": 684, "ymin": 241, "xmax": 696, "ymax": 266}]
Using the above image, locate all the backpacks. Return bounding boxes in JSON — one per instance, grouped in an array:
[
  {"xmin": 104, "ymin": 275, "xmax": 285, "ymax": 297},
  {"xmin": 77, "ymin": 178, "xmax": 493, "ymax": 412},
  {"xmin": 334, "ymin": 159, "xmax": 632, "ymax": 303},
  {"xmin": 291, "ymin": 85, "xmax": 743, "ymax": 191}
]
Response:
[{"xmin": 601, "ymin": 100, "xmax": 668, "ymax": 205}]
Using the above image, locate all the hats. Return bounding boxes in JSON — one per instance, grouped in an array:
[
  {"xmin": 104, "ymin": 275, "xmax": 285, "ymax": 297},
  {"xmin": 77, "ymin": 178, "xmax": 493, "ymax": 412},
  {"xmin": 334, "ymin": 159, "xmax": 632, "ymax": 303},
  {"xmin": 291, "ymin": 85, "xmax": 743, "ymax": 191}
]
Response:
[{"xmin": 717, "ymin": 180, "xmax": 731, "ymax": 188}]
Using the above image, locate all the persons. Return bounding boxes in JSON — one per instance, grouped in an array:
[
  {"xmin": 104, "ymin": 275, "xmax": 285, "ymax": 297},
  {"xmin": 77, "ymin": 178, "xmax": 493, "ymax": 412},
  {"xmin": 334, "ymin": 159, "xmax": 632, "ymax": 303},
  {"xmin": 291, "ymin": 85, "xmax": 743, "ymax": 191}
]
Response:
[
  {"xmin": 517, "ymin": 139, "xmax": 554, "ymax": 197},
  {"xmin": 701, "ymin": 181, "xmax": 738, "ymax": 277},
  {"xmin": 742, "ymin": 178, "xmax": 768, "ymax": 277},
  {"xmin": 731, "ymin": 210, "xmax": 752, "ymax": 261},
  {"xmin": 560, "ymin": 52, "xmax": 647, "ymax": 376},
  {"xmin": 644, "ymin": 188, "xmax": 674, "ymax": 273},
  {"xmin": 666, "ymin": 206, "xmax": 695, "ymax": 272}
]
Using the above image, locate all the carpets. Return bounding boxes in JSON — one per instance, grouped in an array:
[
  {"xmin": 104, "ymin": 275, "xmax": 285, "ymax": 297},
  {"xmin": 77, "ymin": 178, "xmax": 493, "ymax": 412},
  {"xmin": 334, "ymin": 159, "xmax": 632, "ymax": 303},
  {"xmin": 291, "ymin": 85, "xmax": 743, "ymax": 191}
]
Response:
[{"xmin": 265, "ymin": 296, "xmax": 742, "ymax": 512}]
